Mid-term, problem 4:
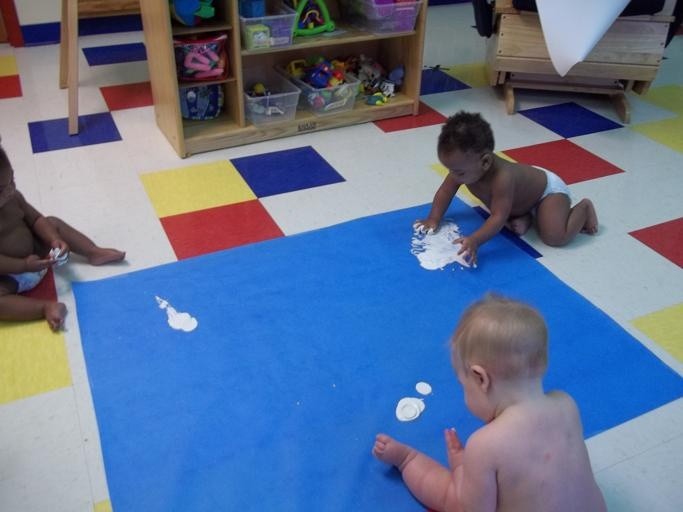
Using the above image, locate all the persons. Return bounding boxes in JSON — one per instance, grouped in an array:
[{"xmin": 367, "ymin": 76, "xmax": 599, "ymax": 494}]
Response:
[
  {"xmin": 412, "ymin": 110, "xmax": 598, "ymax": 266},
  {"xmin": 372, "ymin": 292, "xmax": 608, "ymax": 512},
  {"xmin": 0, "ymin": 147, "xmax": 125, "ymax": 332}
]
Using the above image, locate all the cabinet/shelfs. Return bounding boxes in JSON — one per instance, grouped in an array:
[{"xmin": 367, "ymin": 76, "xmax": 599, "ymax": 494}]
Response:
[{"xmin": 139, "ymin": 0, "xmax": 429, "ymax": 160}]
